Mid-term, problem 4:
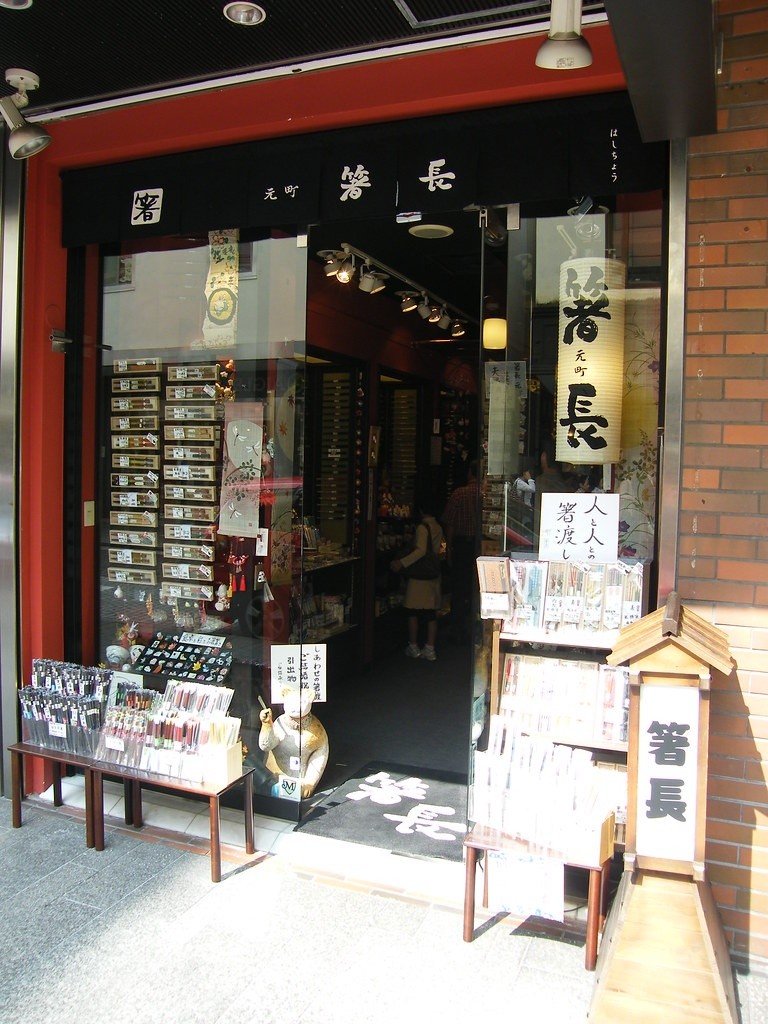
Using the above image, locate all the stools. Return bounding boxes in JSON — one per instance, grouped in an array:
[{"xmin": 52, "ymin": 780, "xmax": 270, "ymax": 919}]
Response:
[
  {"xmin": 9, "ymin": 743, "xmax": 134, "ymax": 848},
  {"xmin": 88, "ymin": 760, "xmax": 256, "ymax": 882},
  {"xmin": 462, "ymin": 823, "xmax": 611, "ymax": 972}
]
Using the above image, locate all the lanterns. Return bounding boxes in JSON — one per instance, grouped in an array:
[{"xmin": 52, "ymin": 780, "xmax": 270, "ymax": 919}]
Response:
[{"xmin": 557, "ymin": 258, "xmax": 626, "ymax": 463}]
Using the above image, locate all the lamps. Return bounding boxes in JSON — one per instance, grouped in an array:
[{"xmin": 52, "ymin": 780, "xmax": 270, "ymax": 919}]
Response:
[
  {"xmin": 535, "ymin": 0, "xmax": 593, "ymax": 70},
  {"xmin": 316, "ymin": 243, "xmax": 466, "ymax": 337},
  {"xmin": 0, "ymin": 69, "xmax": 53, "ymax": 161}
]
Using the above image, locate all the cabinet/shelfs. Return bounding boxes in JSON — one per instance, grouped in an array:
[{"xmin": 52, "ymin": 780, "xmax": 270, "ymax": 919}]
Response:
[
  {"xmin": 293, "ymin": 543, "xmax": 415, "ymax": 644},
  {"xmin": 488, "ymin": 551, "xmax": 655, "ymax": 855}
]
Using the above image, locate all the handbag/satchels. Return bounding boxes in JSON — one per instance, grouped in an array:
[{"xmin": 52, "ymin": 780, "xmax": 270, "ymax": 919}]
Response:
[{"xmin": 408, "ymin": 522, "xmax": 441, "ymax": 579}]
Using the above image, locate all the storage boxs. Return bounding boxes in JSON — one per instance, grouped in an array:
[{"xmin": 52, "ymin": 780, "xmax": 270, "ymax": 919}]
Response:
[
  {"xmin": 467, "ymin": 784, "xmax": 615, "ymax": 866},
  {"xmin": 22, "ymin": 715, "xmax": 242, "ymax": 785},
  {"xmin": 453, "ymin": 633, "xmax": 483, "ymax": 671}
]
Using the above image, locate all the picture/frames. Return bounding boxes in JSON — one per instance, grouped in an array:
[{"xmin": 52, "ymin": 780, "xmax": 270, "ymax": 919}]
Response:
[
  {"xmin": 238, "ymin": 241, "xmax": 257, "ymax": 279},
  {"xmin": 102, "ymin": 253, "xmax": 135, "ymax": 293}
]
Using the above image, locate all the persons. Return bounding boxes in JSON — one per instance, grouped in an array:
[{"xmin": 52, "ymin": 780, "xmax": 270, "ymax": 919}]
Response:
[
  {"xmin": 443, "ymin": 462, "xmax": 482, "ymax": 640},
  {"xmin": 533, "ymin": 442, "xmax": 570, "ymax": 543},
  {"xmin": 512, "ymin": 468, "xmax": 535, "ymax": 506},
  {"xmin": 392, "ymin": 497, "xmax": 444, "ymax": 662}
]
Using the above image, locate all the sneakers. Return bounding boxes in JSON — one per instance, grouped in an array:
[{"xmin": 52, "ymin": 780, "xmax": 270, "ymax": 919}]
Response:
[
  {"xmin": 420, "ymin": 648, "xmax": 436, "ymax": 660},
  {"xmin": 404, "ymin": 642, "xmax": 421, "ymax": 658}
]
